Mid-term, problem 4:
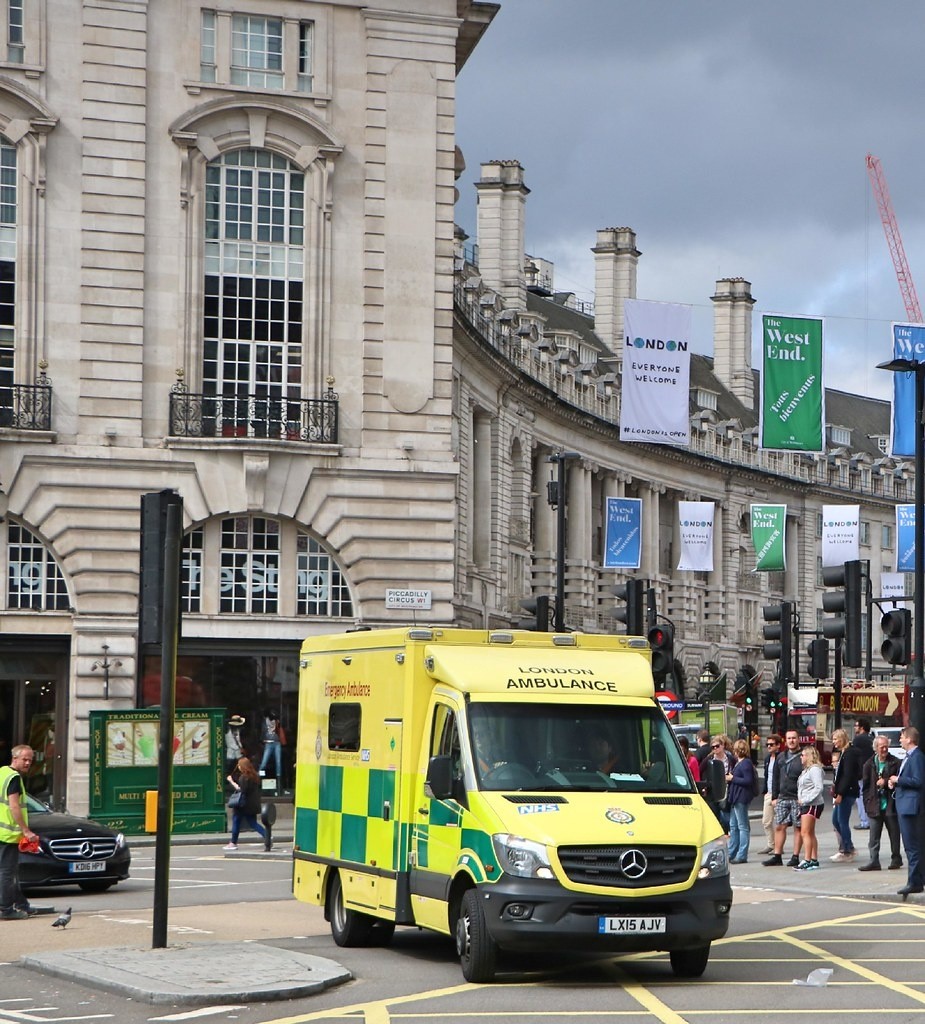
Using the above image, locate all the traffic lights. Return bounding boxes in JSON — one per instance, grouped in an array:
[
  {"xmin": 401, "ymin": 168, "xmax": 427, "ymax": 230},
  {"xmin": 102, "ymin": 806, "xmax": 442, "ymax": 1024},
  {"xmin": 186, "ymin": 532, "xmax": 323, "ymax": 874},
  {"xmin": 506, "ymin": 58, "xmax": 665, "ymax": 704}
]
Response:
[
  {"xmin": 645, "ymin": 624, "xmax": 674, "ymax": 678},
  {"xmin": 807, "ymin": 639, "xmax": 830, "ymax": 679},
  {"xmin": 762, "ymin": 602, "xmax": 793, "ymax": 681},
  {"xmin": 761, "ymin": 687, "xmax": 774, "ymax": 707},
  {"xmin": 880, "ymin": 609, "xmax": 911, "ymax": 665},
  {"xmin": 745, "ymin": 694, "xmax": 758, "ymax": 724},
  {"xmin": 819, "ymin": 561, "xmax": 862, "ymax": 668},
  {"xmin": 606, "ymin": 578, "xmax": 645, "ymax": 635},
  {"xmin": 518, "ymin": 596, "xmax": 549, "ymax": 631},
  {"xmin": 769, "ymin": 695, "xmax": 776, "ymax": 715}
]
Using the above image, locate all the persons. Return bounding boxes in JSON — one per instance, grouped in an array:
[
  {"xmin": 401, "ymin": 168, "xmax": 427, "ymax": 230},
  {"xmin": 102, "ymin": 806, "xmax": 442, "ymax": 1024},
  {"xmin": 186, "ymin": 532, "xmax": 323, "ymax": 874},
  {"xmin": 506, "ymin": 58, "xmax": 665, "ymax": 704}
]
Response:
[
  {"xmin": 858, "ymin": 734, "xmax": 903, "ymax": 872},
  {"xmin": 757, "ymin": 734, "xmax": 785, "ymax": 855},
  {"xmin": 703, "ymin": 736, "xmax": 736, "ymax": 835},
  {"xmin": 829, "ymin": 729, "xmax": 862, "ymax": 863},
  {"xmin": 587, "ymin": 731, "xmax": 626, "ymax": 774},
  {"xmin": 853, "ymin": 718, "xmax": 875, "ymax": 829},
  {"xmin": 43, "ymin": 725, "xmax": 53, "ymax": 806},
  {"xmin": 751, "ymin": 730, "xmax": 760, "ymax": 766},
  {"xmin": 454, "ymin": 725, "xmax": 508, "ymax": 783},
  {"xmin": 761, "ymin": 730, "xmax": 804, "ymax": 867},
  {"xmin": 257, "ymin": 709, "xmax": 291, "ymax": 794},
  {"xmin": 225, "ymin": 715, "xmax": 245, "ymax": 773},
  {"xmin": 677, "ymin": 736, "xmax": 700, "ymax": 785},
  {"xmin": 740, "ymin": 726, "xmax": 749, "ymax": 741},
  {"xmin": 727, "ymin": 738, "xmax": 758, "ymax": 864},
  {"xmin": 792, "ymin": 746, "xmax": 825, "ymax": 871},
  {"xmin": 223, "ymin": 757, "xmax": 272, "ymax": 851},
  {"xmin": 642, "ymin": 739, "xmax": 669, "ymax": 784},
  {"xmin": 694, "ymin": 729, "xmax": 713, "ymax": 762},
  {"xmin": 0, "ymin": 745, "xmax": 53, "ymax": 919},
  {"xmin": 888, "ymin": 727, "xmax": 925, "ymax": 895}
]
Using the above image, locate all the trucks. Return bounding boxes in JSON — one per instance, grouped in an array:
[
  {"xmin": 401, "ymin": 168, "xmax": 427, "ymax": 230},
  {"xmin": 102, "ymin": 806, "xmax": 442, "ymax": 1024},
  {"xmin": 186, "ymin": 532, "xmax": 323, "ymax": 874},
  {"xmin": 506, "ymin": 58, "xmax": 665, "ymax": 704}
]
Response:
[{"xmin": 787, "ymin": 681, "xmax": 835, "ymax": 750}]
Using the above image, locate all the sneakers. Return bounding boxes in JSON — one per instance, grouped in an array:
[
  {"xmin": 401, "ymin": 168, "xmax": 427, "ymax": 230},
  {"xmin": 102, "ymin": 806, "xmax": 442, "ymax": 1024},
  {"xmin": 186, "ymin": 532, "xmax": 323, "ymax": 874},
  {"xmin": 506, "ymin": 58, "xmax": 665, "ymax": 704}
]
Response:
[
  {"xmin": 222, "ymin": 843, "xmax": 238, "ymax": 850},
  {"xmin": 811, "ymin": 860, "xmax": 819, "ymax": 868},
  {"xmin": 793, "ymin": 860, "xmax": 812, "ymax": 871}
]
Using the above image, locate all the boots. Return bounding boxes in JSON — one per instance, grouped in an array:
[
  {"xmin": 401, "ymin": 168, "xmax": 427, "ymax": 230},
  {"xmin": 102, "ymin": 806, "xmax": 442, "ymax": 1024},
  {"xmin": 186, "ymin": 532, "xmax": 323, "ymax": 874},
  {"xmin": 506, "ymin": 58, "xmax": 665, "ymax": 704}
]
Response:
[{"xmin": 277, "ymin": 776, "xmax": 282, "ymax": 797}]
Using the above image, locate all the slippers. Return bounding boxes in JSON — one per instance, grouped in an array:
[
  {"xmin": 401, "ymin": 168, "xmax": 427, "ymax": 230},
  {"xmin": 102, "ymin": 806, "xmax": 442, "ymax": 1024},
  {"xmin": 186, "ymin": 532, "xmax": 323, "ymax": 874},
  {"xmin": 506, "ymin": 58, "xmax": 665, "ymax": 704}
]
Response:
[{"xmin": 765, "ymin": 743, "xmax": 776, "ymax": 747}]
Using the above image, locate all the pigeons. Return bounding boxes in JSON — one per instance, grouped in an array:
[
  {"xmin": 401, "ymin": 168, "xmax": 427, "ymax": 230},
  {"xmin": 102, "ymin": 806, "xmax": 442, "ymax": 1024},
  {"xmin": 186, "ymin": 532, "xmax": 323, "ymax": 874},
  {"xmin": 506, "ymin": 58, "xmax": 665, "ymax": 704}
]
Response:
[{"xmin": 51, "ymin": 907, "xmax": 72, "ymax": 930}]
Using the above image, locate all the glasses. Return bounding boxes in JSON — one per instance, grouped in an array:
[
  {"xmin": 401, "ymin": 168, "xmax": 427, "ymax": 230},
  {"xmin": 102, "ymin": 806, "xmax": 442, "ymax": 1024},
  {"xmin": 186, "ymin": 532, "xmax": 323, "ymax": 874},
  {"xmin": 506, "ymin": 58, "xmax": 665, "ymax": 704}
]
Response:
[
  {"xmin": 801, "ymin": 753, "xmax": 807, "ymax": 757},
  {"xmin": 710, "ymin": 744, "xmax": 721, "ymax": 748}
]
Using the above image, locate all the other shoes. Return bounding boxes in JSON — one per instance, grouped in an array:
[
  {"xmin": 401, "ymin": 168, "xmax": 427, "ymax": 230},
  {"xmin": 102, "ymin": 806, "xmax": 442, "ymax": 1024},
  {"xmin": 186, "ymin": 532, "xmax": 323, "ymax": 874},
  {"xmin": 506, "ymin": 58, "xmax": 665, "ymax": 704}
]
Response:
[
  {"xmin": 761, "ymin": 856, "xmax": 783, "ymax": 865},
  {"xmin": 858, "ymin": 864, "xmax": 880, "ymax": 870},
  {"xmin": 830, "ymin": 853, "xmax": 852, "ymax": 862},
  {"xmin": 731, "ymin": 858, "xmax": 747, "ymax": 863},
  {"xmin": 757, "ymin": 846, "xmax": 773, "ymax": 854},
  {"xmin": 0, "ymin": 906, "xmax": 38, "ymax": 920},
  {"xmin": 888, "ymin": 858, "xmax": 903, "ymax": 869},
  {"xmin": 845, "ymin": 850, "xmax": 858, "ymax": 861},
  {"xmin": 853, "ymin": 824, "xmax": 870, "ymax": 830},
  {"xmin": 897, "ymin": 884, "xmax": 924, "ymax": 894},
  {"xmin": 787, "ymin": 858, "xmax": 799, "ymax": 866},
  {"xmin": 769, "ymin": 849, "xmax": 784, "ymax": 854}
]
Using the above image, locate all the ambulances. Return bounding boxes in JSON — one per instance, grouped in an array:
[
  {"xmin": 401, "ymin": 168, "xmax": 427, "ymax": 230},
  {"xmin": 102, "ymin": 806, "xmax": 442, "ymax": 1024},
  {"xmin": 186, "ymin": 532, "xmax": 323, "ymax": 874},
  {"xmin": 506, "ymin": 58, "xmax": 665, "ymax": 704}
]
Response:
[{"xmin": 291, "ymin": 625, "xmax": 733, "ymax": 985}]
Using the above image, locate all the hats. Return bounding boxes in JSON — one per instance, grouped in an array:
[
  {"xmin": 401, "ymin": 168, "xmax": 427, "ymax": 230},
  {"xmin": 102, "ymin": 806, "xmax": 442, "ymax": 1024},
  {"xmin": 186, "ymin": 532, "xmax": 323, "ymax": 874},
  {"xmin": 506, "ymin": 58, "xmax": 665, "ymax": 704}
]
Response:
[{"xmin": 225, "ymin": 715, "xmax": 246, "ymax": 725}]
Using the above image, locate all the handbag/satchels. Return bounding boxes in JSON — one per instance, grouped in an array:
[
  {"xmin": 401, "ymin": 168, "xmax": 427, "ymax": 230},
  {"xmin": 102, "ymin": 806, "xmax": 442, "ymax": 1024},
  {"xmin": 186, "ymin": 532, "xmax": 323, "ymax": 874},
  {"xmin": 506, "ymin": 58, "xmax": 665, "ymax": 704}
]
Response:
[
  {"xmin": 277, "ymin": 720, "xmax": 287, "ymax": 746},
  {"xmin": 241, "ymin": 747, "xmax": 248, "ymax": 754},
  {"xmin": 227, "ymin": 785, "xmax": 247, "ymax": 809}
]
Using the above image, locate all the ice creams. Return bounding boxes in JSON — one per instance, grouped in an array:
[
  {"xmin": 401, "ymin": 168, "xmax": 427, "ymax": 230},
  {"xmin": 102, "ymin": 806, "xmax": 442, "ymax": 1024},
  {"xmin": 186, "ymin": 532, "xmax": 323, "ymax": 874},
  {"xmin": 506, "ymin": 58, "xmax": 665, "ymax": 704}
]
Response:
[
  {"xmin": 112, "ymin": 730, "xmax": 127, "ymax": 758},
  {"xmin": 191, "ymin": 728, "xmax": 207, "ymax": 759}
]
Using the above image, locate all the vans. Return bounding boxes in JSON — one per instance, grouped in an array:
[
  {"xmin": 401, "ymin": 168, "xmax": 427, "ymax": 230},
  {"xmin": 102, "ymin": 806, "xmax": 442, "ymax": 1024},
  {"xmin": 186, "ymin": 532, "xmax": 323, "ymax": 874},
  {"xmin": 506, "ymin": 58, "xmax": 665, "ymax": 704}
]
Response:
[
  {"xmin": 670, "ymin": 724, "xmax": 703, "ymax": 754},
  {"xmin": 867, "ymin": 725, "xmax": 908, "ymax": 761}
]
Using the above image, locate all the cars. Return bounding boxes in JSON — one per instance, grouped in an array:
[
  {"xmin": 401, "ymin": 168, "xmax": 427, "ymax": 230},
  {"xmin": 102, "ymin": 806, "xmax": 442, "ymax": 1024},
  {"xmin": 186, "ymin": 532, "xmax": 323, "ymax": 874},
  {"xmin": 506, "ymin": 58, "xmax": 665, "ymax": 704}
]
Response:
[{"xmin": 15, "ymin": 790, "xmax": 131, "ymax": 899}]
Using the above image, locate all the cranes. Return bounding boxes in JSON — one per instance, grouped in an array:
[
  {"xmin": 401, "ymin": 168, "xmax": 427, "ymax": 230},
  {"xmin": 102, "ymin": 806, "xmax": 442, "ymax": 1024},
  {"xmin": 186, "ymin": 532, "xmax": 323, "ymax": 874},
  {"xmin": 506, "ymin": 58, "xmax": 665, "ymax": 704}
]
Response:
[{"xmin": 864, "ymin": 152, "xmax": 923, "ymax": 323}]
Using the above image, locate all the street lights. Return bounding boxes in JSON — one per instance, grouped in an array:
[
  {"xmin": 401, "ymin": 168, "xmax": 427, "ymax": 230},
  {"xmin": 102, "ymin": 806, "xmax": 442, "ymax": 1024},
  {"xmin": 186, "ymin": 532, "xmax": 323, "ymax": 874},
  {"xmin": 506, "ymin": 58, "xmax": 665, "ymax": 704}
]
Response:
[
  {"xmin": 876, "ymin": 359, "xmax": 924, "ymax": 752},
  {"xmin": 546, "ymin": 446, "xmax": 581, "ymax": 632}
]
